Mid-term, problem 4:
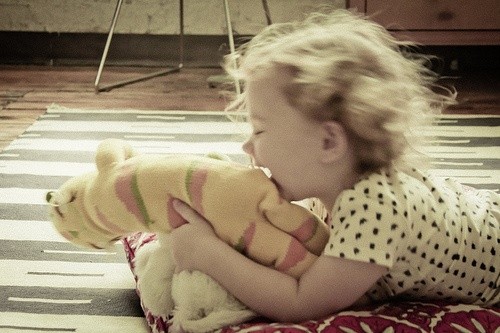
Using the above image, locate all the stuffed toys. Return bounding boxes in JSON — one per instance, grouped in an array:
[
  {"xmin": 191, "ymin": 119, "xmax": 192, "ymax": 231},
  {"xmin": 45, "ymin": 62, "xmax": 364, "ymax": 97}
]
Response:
[{"xmin": 45, "ymin": 137, "xmax": 333, "ymax": 279}]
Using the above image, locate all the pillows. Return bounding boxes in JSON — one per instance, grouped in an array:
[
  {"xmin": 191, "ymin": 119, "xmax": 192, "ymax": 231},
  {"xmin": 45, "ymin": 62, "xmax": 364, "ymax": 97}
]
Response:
[{"xmin": 122, "ymin": 230, "xmax": 500, "ymax": 332}]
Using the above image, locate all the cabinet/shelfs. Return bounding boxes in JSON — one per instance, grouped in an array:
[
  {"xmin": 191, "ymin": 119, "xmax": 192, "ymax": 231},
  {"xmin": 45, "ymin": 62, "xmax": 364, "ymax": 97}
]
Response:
[{"xmin": 346, "ymin": 0, "xmax": 500, "ymax": 50}]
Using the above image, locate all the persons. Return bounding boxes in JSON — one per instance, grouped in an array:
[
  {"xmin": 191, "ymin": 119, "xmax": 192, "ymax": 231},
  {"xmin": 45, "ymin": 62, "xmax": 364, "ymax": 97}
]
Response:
[{"xmin": 159, "ymin": 8, "xmax": 499, "ymax": 324}]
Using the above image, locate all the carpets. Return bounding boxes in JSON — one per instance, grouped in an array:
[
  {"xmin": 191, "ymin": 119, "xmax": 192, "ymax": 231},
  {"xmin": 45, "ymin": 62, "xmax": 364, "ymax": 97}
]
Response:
[{"xmin": 0, "ymin": 100, "xmax": 499, "ymax": 332}]
